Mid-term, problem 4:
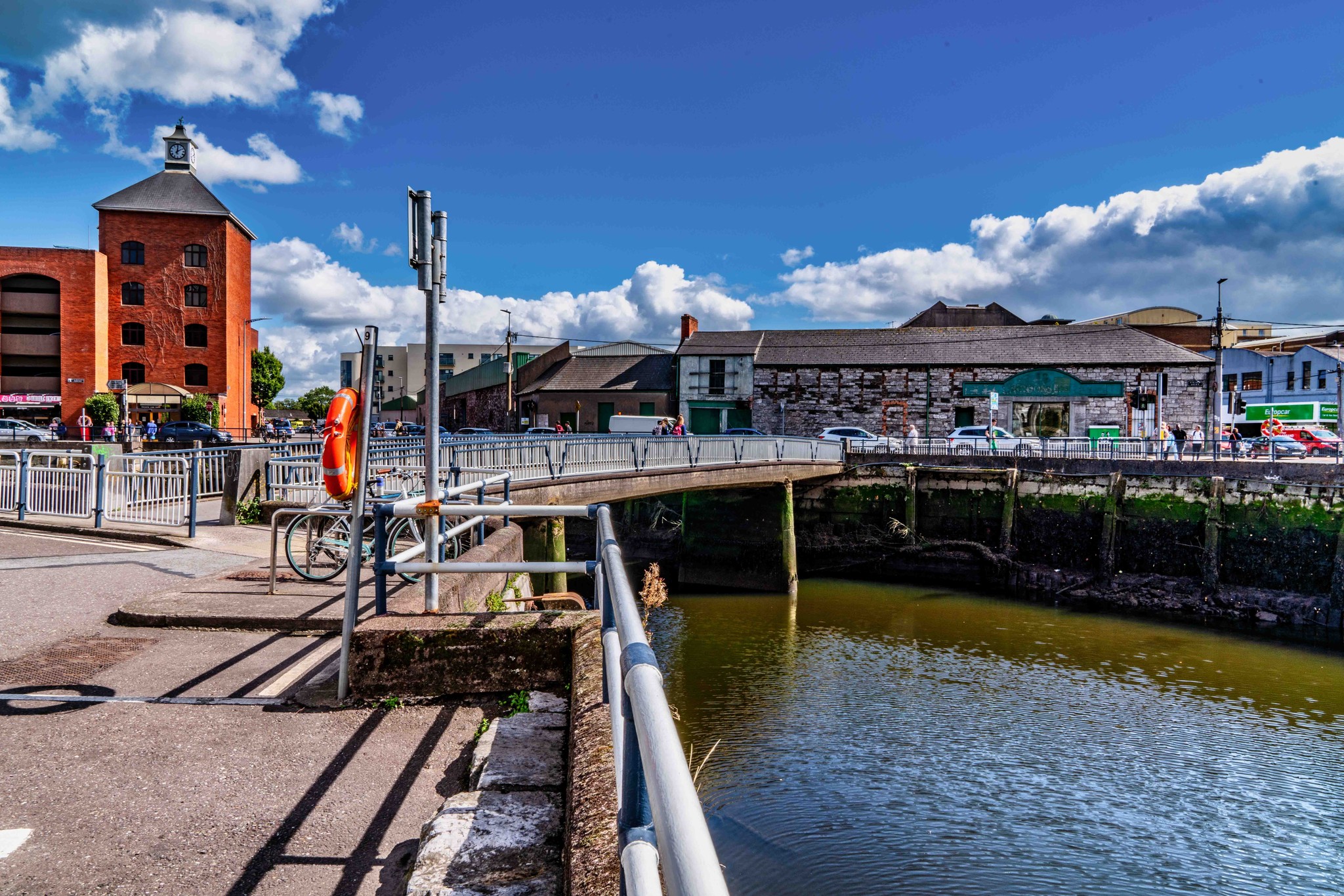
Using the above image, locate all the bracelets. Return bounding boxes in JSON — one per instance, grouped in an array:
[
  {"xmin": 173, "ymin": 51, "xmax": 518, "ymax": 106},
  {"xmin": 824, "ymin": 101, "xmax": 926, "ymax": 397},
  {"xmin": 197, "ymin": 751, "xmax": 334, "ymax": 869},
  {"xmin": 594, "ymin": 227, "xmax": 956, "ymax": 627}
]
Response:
[{"xmin": 1202, "ymin": 445, "xmax": 1204, "ymax": 446}]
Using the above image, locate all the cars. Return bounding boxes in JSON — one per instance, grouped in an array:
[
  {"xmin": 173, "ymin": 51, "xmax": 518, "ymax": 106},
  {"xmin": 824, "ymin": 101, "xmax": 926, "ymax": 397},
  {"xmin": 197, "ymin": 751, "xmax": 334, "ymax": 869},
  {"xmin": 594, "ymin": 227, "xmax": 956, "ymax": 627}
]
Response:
[
  {"xmin": 252, "ymin": 421, "xmax": 270, "ymax": 438},
  {"xmin": 522, "ymin": 427, "xmax": 565, "ymax": 444},
  {"xmin": 453, "ymin": 428, "xmax": 498, "ymax": 447},
  {"xmin": 273, "ymin": 418, "xmax": 293, "ymax": 439},
  {"xmin": 291, "ymin": 427, "xmax": 295, "ymax": 436},
  {"xmin": 295, "ymin": 426, "xmax": 324, "ymax": 434},
  {"xmin": 0, "ymin": 419, "xmax": 59, "ymax": 442},
  {"xmin": 371, "ymin": 422, "xmax": 422, "ymax": 447},
  {"xmin": 1220, "ymin": 427, "xmax": 1344, "ymax": 460},
  {"xmin": 415, "ymin": 426, "xmax": 453, "ymax": 445},
  {"xmin": 722, "ymin": 428, "xmax": 769, "ymax": 437}
]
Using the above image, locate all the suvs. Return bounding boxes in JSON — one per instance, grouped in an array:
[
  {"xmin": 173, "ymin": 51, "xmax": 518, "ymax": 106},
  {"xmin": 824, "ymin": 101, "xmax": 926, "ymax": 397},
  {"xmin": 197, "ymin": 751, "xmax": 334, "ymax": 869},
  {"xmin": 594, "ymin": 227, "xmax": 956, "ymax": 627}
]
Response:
[
  {"xmin": 817, "ymin": 426, "xmax": 904, "ymax": 453},
  {"xmin": 157, "ymin": 421, "xmax": 234, "ymax": 444},
  {"xmin": 944, "ymin": 425, "xmax": 1042, "ymax": 457}
]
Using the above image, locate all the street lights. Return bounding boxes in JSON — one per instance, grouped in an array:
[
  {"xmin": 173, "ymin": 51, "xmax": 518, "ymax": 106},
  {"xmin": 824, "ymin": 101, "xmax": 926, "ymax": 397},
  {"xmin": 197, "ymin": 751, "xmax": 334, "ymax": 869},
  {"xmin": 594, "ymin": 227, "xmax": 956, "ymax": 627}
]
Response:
[
  {"xmin": 375, "ymin": 381, "xmax": 381, "ymax": 422},
  {"xmin": 242, "ymin": 317, "xmax": 273, "ymax": 441},
  {"xmin": 500, "ymin": 310, "xmax": 511, "ymax": 432},
  {"xmin": 1213, "ymin": 277, "xmax": 1229, "ymax": 458},
  {"xmin": 396, "ymin": 377, "xmax": 403, "ymax": 422}
]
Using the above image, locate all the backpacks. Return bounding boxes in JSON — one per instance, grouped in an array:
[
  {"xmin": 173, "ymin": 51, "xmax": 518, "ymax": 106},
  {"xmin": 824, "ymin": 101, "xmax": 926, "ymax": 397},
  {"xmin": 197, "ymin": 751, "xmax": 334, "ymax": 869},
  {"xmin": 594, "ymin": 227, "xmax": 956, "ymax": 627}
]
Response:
[
  {"xmin": 652, "ymin": 423, "xmax": 666, "ymax": 436},
  {"xmin": 397, "ymin": 423, "xmax": 403, "ymax": 432},
  {"xmin": 673, "ymin": 424, "xmax": 685, "ymax": 439}
]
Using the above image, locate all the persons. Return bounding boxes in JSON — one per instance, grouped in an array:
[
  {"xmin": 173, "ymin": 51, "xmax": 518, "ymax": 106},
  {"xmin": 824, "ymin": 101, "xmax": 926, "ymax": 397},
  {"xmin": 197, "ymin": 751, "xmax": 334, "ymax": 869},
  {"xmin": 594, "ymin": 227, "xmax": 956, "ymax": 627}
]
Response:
[
  {"xmin": 855, "ymin": 431, "xmax": 869, "ymax": 437},
  {"xmin": 393, "ymin": 419, "xmax": 405, "ymax": 443},
  {"xmin": 136, "ymin": 421, "xmax": 147, "ymax": 442},
  {"xmin": 904, "ymin": 424, "xmax": 918, "ymax": 454},
  {"xmin": 146, "ymin": 420, "xmax": 157, "ymax": 441},
  {"xmin": 565, "ymin": 421, "xmax": 572, "ymax": 437},
  {"xmin": 48, "ymin": 419, "xmax": 67, "ymax": 435},
  {"xmin": 1172, "ymin": 423, "xmax": 1187, "ymax": 460},
  {"xmin": 658, "ymin": 418, "xmax": 671, "ymax": 435},
  {"xmin": 1228, "ymin": 428, "xmax": 1243, "ymax": 461},
  {"xmin": 101, "ymin": 421, "xmax": 117, "ymax": 442},
  {"xmin": 552, "ymin": 421, "xmax": 564, "ymax": 437},
  {"xmin": 312, "ymin": 424, "xmax": 317, "ymax": 435},
  {"xmin": 1155, "ymin": 421, "xmax": 1180, "ymax": 460},
  {"xmin": 42, "ymin": 421, "xmax": 46, "ymax": 426},
  {"xmin": 672, "ymin": 414, "xmax": 687, "ymax": 435},
  {"xmin": 118, "ymin": 418, "xmax": 136, "ymax": 442},
  {"xmin": 985, "ymin": 419, "xmax": 998, "ymax": 456},
  {"xmin": 1190, "ymin": 425, "xmax": 1204, "ymax": 461},
  {"xmin": 260, "ymin": 421, "xmax": 275, "ymax": 443}
]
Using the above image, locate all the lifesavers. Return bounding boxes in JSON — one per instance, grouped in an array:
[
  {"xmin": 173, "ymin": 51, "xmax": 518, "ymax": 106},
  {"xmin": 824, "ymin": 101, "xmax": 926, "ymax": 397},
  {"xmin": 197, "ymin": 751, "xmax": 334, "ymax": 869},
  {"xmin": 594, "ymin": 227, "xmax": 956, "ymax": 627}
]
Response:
[
  {"xmin": 321, "ymin": 388, "xmax": 360, "ymax": 500},
  {"xmin": 78, "ymin": 415, "xmax": 92, "ymax": 428},
  {"xmin": 1260, "ymin": 418, "xmax": 1283, "ymax": 437}
]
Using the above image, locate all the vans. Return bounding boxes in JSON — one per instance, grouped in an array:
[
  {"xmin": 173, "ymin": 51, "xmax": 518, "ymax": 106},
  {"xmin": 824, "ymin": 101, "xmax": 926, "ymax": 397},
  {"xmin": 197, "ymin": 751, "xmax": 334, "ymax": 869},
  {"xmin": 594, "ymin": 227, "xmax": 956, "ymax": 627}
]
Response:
[
  {"xmin": 608, "ymin": 412, "xmax": 694, "ymax": 450},
  {"xmin": 291, "ymin": 421, "xmax": 304, "ymax": 429}
]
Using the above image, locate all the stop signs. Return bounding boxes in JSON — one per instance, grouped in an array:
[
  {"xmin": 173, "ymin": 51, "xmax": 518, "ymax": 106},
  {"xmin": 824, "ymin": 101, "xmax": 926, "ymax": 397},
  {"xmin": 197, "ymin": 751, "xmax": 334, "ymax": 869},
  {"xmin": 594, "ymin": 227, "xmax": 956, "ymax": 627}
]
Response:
[{"xmin": 206, "ymin": 401, "xmax": 214, "ymax": 412}]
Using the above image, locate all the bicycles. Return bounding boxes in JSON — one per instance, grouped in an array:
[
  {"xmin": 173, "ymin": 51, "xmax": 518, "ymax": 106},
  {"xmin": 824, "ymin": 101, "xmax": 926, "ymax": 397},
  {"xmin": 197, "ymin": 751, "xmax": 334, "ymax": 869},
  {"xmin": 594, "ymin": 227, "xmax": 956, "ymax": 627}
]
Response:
[{"xmin": 283, "ymin": 466, "xmax": 478, "ymax": 585}]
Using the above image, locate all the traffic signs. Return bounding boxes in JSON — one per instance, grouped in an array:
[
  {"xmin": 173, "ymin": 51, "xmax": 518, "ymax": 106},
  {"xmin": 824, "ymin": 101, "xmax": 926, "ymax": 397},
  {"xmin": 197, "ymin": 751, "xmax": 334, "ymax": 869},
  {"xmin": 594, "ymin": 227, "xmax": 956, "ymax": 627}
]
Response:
[{"xmin": 106, "ymin": 380, "xmax": 125, "ymax": 389}]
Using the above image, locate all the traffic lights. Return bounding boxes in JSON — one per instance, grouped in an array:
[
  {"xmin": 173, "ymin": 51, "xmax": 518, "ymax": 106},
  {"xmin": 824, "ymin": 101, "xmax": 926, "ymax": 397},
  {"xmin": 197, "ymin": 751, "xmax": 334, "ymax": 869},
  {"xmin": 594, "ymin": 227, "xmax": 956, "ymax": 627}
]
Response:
[
  {"xmin": 1138, "ymin": 393, "xmax": 1148, "ymax": 411},
  {"xmin": 1234, "ymin": 399, "xmax": 1247, "ymax": 415},
  {"xmin": 1130, "ymin": 387, "xmax": 1139, "ymax": 410}
]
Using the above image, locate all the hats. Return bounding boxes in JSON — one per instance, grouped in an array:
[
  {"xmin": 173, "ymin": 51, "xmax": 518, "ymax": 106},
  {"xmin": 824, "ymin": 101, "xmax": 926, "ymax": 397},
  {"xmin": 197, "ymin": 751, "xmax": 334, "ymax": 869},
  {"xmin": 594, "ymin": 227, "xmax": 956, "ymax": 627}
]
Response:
[{"xmin": 395, "ymin": 419, "xmax": 399, "ymax": 421}]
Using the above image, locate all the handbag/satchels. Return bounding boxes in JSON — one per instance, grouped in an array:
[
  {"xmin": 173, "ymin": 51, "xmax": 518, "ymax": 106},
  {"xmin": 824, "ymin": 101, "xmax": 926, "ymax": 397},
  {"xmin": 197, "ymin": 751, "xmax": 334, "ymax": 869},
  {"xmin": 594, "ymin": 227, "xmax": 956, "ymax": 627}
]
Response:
[
  {"xmin": 142, "ymin": 434, "xmax": 146, "ymax": 440},
  {"xmin": 1149, "ymin": 429, "xmax": 1158, "ymax": 443},
  {"xmin": 1236, "ymin": 442, "xmax": 1242, "ymax": 448},
  {"xmin": 260, "ymin": 435, "xmax": 264, "ymax": 442},
  {"xmin": 151, "ymin": 434, "xmax": 155, "ymax": 439},
  {"xmin": 991, "ymin": 440, "xmax": 997, "ymax": 449}
]
